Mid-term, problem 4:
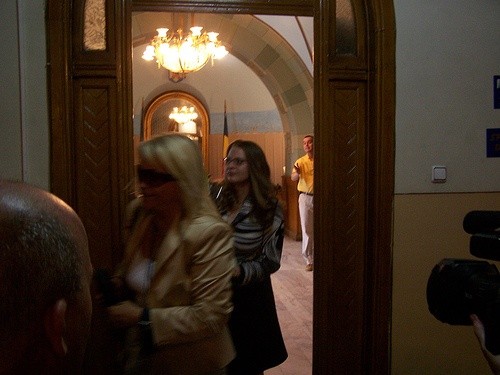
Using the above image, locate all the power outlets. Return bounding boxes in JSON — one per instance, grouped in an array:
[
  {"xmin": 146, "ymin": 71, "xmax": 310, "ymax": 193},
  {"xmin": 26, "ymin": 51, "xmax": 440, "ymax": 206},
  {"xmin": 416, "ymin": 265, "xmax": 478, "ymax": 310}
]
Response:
[{"xmin": 432, "ymin": 167, "xmax": 448, "ymax": 184}]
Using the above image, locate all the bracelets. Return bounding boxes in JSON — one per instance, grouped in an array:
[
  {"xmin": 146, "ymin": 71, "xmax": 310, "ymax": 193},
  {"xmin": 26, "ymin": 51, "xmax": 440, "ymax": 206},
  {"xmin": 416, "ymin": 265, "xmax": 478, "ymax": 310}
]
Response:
[{"xmin": 137, "ymin": 308, "xmax": 151, "ymax": 331}]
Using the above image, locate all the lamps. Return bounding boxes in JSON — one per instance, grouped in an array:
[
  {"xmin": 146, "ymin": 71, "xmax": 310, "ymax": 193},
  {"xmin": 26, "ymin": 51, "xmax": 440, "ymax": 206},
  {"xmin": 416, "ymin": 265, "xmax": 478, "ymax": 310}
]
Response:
[
  {"xmin": 140, "ymin": 10, "xmax": 230, "ymax": 82},
  {"xmin": 169, "ymin": 106, "xmax": 199, "ymax": 135}
]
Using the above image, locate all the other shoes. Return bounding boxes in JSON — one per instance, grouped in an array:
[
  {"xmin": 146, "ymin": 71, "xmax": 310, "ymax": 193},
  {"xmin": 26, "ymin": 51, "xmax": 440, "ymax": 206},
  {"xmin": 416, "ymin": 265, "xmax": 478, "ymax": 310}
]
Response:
[{"xmin": 304, "ymin": 264, "xmax": 312, "ymax": 271}]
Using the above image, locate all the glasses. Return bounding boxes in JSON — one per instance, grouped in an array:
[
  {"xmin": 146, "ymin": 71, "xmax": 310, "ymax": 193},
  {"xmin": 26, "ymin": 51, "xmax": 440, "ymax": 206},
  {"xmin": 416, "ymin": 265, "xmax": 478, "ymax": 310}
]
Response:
[
  {"xmin": 223, "ymin": 157, "xmax": 247, "ymax": 166},
  {"xmin": 137, "ymin": 168, "xmax": 174, "ymax": 187}
]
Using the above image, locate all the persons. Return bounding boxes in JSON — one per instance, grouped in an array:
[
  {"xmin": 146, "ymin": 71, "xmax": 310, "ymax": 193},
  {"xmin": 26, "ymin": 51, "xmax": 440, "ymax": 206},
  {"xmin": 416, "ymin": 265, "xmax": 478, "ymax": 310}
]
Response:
[
  {"xmin": 470, "ymin": 314, "xmax": 500, "ymax": 375},
  {"xmin": 0, "ymin": 179, "xmax": 93, "ymax": 375},
  {"xmin": 107, "ymin": 135, "xmax": 237, "ymax": 375},
  {"xmin": 212, "ymin": 139, "xmax": 288, "ymax": 375},
  {"xmin": 291, "ymin": 135, "xmax": 314, "ymax": 271}
]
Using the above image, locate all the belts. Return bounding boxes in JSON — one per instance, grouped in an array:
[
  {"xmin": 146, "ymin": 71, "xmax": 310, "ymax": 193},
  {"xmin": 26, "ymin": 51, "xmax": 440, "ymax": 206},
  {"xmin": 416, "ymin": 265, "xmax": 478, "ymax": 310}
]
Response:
[{"xmin": 300, "ymin": 191, "xmax": 313, "ymax": 196}]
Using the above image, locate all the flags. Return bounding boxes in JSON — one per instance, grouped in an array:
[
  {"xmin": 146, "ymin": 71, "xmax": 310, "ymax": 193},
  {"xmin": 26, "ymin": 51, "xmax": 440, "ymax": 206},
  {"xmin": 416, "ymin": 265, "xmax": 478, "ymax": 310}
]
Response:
[{"xmin": 222, "ymin": 113, "xmax": 229, "ymax": 175}]
